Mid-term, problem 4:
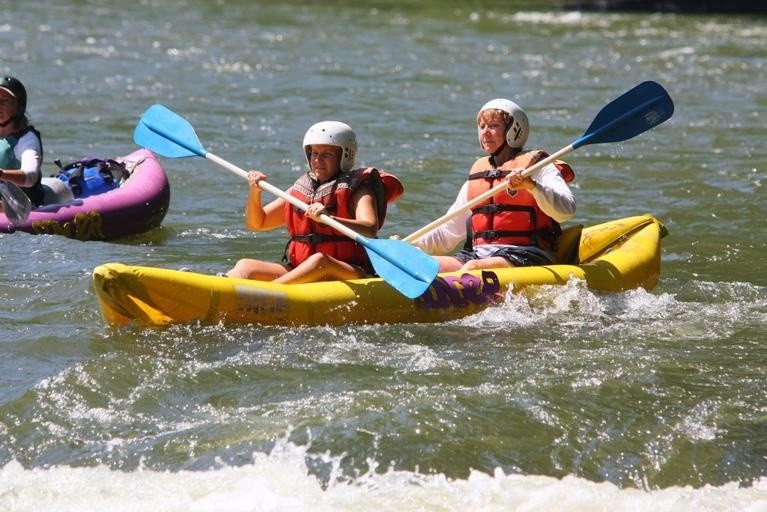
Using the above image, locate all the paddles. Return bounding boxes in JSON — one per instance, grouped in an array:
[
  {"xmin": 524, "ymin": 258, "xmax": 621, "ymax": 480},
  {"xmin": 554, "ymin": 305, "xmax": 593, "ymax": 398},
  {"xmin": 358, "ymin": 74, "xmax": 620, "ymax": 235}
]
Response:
[
  {"xmin": 133, "ymin": 104, "xmax": 439, "ymax": 298},
  {"xmin": 402, "ymin": 80, "xmax": 674, "ymax": 249},
  {"xmin": 0, "ymin": 180, "xmax": 32, "ymax": 224}
]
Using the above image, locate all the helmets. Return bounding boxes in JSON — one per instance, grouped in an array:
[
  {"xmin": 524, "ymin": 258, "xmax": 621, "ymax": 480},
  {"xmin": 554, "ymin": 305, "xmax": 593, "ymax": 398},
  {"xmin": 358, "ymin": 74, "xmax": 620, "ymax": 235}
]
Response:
[
  {"xmin": 302, "ymin": 121, "xmax": 357, "ymax": 172},
  {"xmin": 0, "ymin": 74, "xmax": 27, "ymax": 119},
  {"xmin": 477, "ymin": 99, "xmax": 529, "ymax": 153}
]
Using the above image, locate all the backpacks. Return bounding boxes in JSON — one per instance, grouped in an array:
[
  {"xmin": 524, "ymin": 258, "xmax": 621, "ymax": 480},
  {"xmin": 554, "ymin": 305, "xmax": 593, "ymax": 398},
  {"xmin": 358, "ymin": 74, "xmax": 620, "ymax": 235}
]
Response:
[{"xmin": 56, "ymin": 157, "xmax": 120, "ymax": 198}]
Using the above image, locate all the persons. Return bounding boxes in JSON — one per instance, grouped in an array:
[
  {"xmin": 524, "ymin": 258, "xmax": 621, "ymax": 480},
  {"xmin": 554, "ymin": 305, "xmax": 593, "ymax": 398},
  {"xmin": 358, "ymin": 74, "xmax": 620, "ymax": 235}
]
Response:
[
  {"xmin": 391, "ymin": 97, "xmax": 576, "ymax": 272},
  {"xmin": 228, "ymin": 119, "xmax": 380, "ymax": 286},
  {"xmin": 0, "ymin": 71, "xmax": 46, "ymax": 211}
]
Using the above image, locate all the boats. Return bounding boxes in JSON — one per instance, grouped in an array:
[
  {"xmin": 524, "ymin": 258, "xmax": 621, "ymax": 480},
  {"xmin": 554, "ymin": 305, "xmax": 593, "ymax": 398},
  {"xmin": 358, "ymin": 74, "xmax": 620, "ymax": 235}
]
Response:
[
  {"xmin": 91, "ymin": 213, "xmax": 671, "ymax": 326},
  {"xmin": 1, "ymin": 149, "xmax": 171, "ymax": 241}
]
